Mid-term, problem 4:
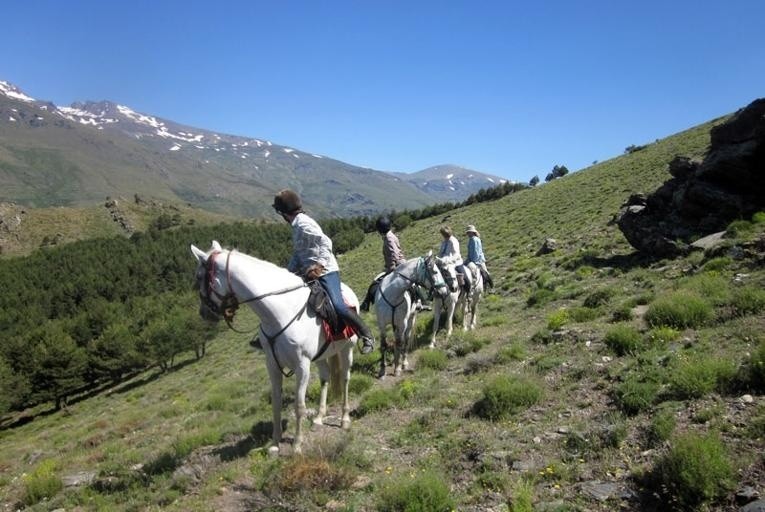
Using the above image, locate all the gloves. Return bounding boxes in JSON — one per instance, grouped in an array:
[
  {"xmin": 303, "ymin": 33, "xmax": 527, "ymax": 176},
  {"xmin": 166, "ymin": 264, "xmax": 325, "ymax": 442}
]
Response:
[{"xmin": 308, "ymin": 265, "xmax": 323, "ymax": 280}]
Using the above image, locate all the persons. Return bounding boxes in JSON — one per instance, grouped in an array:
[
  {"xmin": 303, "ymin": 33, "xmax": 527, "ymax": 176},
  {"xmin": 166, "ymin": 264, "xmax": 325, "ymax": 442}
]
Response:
[
  {"xmin": 358, "ymin": 214, "xmax": 431, "ymax": 314},
  {"xmin": 461, "ymin": 224, "xmax": 496, "ymax": 295},
  {"xmin": 249, "ymin": 189, "xmax": 374, "ymax": 356},
  {"xmin": 437, "ymin": 225, "xmax": 472, "ymax": 294}
]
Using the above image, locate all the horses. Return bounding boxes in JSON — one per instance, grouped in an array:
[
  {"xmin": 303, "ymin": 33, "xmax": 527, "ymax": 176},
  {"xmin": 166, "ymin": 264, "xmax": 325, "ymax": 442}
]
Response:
[
  {"xmin": 372, "ymin": 249, "xmax": 449, "ymax": 381},
  {"xmin": 461, "ymin": 262, "xmax": 490, "ymax": 332},
  {"xmin": 429, "ymin": 253, "xmax": 473, "ymax": 348},
  {"xmin": 190, "ymin": 239, "xmax": 361, "ymax": 453}
]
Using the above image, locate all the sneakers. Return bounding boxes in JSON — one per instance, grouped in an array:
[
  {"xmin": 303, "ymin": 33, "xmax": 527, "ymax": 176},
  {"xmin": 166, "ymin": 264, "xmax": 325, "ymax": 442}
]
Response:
[{"xmin": 361, "ymin": 340, "xmax": 374, "ymax": 354}]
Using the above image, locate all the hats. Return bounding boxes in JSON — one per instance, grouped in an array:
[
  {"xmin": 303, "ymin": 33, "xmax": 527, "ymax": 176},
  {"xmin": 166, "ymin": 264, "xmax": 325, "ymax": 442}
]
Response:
[{"xmin": 465, "ymin": 225, "xmax": 479, "ymax": 235}]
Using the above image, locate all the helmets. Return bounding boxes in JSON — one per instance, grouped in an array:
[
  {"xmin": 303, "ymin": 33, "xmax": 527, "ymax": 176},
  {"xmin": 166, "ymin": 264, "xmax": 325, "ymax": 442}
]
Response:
[
  {"xmin": 272, "ymin": 189, "xmax": 302, "ymax": 212},
  {"xmin": 376, "ymin": 217, "xmax": 392, "ymax": 232}
]
